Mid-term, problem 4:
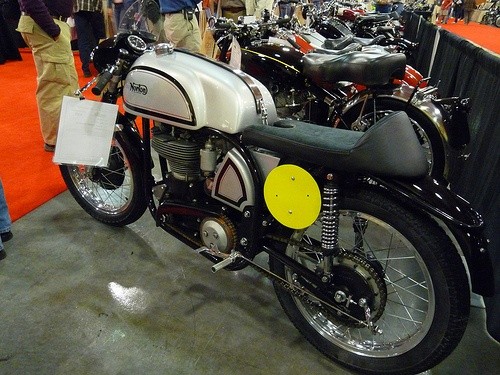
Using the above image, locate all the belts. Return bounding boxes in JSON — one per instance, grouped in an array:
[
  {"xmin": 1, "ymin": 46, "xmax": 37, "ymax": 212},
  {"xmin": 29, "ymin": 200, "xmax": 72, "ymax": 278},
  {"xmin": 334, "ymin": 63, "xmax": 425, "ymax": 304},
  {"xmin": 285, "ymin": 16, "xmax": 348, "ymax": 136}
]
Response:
[
  {"xmin": 165, "ymin": 10, "xmax": 181, "ymax": 14},
  {"xmin": 222, "ymin": 6, "xmax": 244, "ymax": 11},
  {"xmin": 22, "ymin": 11, "xmax": 67, "ymax": 22}
]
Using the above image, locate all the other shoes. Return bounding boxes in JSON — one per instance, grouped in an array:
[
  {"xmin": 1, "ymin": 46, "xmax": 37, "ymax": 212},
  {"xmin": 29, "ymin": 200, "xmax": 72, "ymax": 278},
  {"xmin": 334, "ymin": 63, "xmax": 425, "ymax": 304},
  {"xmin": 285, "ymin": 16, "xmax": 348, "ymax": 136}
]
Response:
[
  {"xmin": 0, "ymin": 243, "xmax": 6, "ymax": 261},
  {"xmin": 436, "ymin": 16, "xmax": 468, "ymax": 25},
  {"xmin": 0, "ymin": 230, "xmax": 13, "ymax": 242},
  {"xmin": 44, "ymin": 143, "xmax": 56, "ymax": 151},
  {"xmin": 83, "ymin": 68, "xmax": 91, "ymax": 77}
]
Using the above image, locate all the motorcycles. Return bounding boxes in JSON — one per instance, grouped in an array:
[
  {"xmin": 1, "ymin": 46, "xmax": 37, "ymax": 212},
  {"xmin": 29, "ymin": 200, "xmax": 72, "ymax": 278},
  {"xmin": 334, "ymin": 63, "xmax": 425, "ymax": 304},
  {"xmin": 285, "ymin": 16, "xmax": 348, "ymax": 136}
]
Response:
[
  {"xmin": 481, "ymin": 0, "xmax": 500, "ymax": 28},
  {"xmin": 55, "ymin": 0, "xmax": 485, "ymax": 375},
  {"xmin": 207, "ymin": 0, "xmax": 472, "ymax": 187}
]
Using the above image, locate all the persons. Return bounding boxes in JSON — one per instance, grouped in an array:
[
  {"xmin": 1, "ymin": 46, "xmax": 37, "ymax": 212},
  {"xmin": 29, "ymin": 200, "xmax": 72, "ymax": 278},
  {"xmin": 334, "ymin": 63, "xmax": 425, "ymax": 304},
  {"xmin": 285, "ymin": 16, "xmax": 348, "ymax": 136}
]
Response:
[
  {"xmin": 407, "ymin": 0, "xmax": 476, "ymax": 25},
  {"xmin": 0, "ymin": 177, "xmax": 13, "ymax": 261},
  {"xmin": 15, "ymin": 0, "xmax": 298, "ymax": 152}
]
